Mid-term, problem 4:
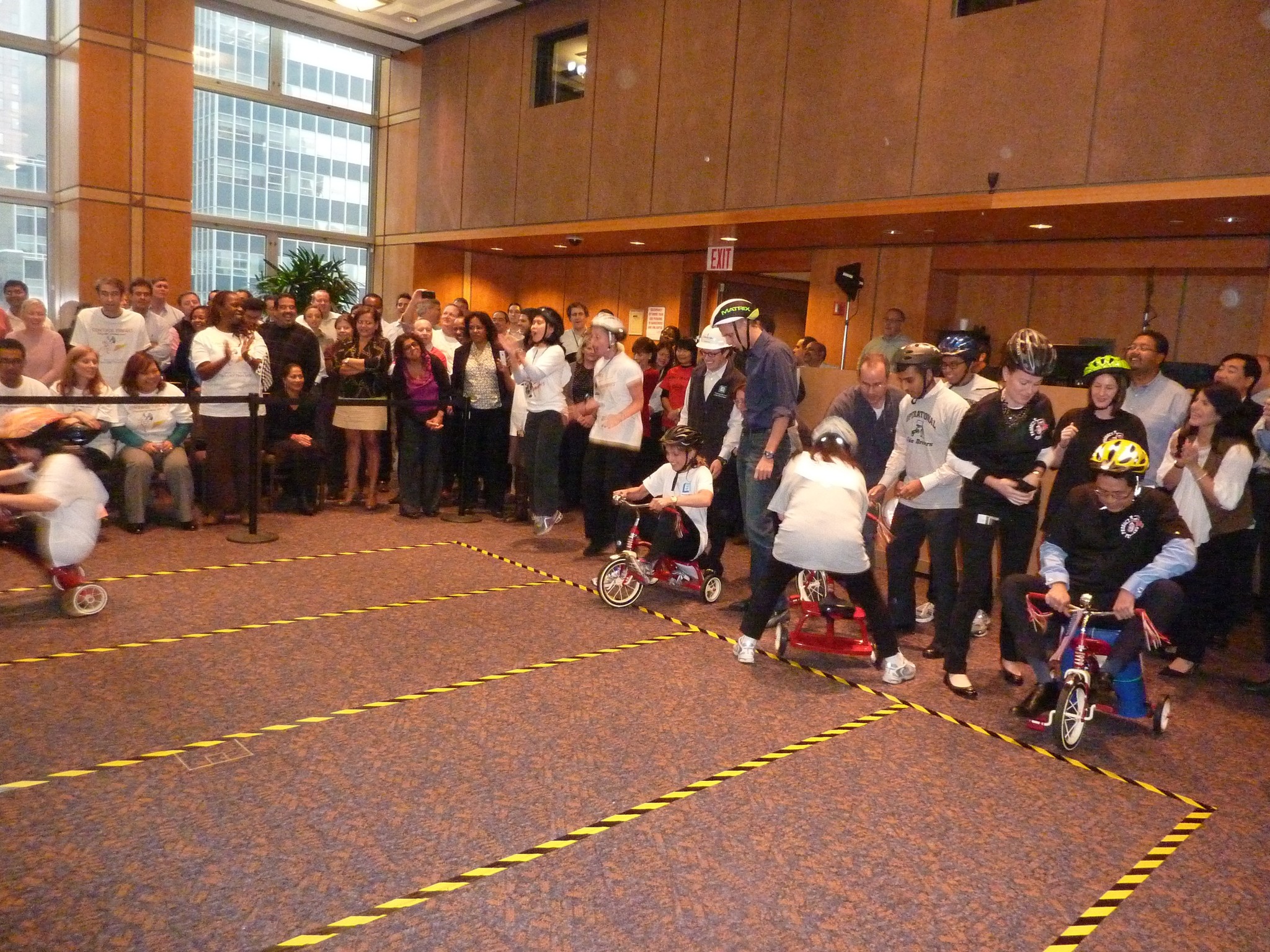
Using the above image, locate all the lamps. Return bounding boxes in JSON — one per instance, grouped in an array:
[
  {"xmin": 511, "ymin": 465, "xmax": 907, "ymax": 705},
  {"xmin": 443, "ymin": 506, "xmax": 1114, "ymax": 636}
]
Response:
[{"xmin": 329, "ymin": 0, "xmax": 396, "ymax": 13}]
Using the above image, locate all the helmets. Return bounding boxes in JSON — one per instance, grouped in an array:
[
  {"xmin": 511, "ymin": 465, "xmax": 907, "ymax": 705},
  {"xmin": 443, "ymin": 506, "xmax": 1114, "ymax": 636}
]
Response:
[
  {"xmin": 810, "ymin": 415, "xmax": 858, "ymax": 457},
  {"xmin": 592, "ymin": 312, "xmax": 626, "ymax": 341},
  {"xmin": 1090, "ymin": 439, "xmax": 1150, "ymax": 474},
  {"xmin": 710, "ymin": 299, "xmax": 759, "ymax": 329},
  {"xmin": 659, "ymin": 425, "xmax": 703, "ymax": 448},
  {"xmin": 893, "ymin": 342, "xmax": 941, "ymax": 363},
  {"xmin": 938, "ymin": 331, "xmax": 976, "ymax": 354},
  {"xmin": 1082, "ymin": 355, "xmax": 1132, "ymax": 381},
  {"xmin": 0, "ymin": 407, "xmax": 61, "ymax": 440},
  {"xmin": 538, "ymin": 307, "xmax": 564, "ymax": 337},
  {"xmin": 1008, "ymin": 328, "xmax": 1053, "ymax": 376},
  {"xmin": 696, "ymin": 324, "xmax": 733, "ymax": 350}
]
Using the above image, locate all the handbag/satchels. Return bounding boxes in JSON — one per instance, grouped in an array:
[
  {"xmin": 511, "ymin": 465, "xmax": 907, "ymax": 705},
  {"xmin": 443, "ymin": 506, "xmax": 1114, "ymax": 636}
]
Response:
[{"xmin": 57, "ymin": 419, "xmax": 114, "ymax": 442}]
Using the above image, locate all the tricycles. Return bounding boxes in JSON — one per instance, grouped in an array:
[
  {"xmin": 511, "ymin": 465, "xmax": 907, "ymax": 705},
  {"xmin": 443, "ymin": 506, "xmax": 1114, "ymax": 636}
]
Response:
[
  {"xmin": 0, "ymin": 510, "xmax": 106, "ymax": 616},
  {"xmin": 596, "ymin": 495, "xmax": 724, "ymax": 608},
  {"xmin": 1023, "ymin": 588, "xmax": 1179, "ymax": 752},
  {"xmin": 773, "ymin": 570, "xmax": 880, "ymax": 665}
]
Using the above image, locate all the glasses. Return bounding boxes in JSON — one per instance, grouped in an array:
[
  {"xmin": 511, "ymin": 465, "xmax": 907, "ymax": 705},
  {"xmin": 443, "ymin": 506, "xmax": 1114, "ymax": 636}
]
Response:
[
  {"xmin": 882, "ymin": 317, "xmax": 903, "ymax": 324},
  {"xmin": 699, "ymin": 349, "xmax": 725, "ymax": 358},
  {"xmin": 1094, "ymin": 484, "xmax": 1135, "ymax": 499},
  {"xmin": 1128, "ymin": 343, "xmax": 1162, "ymax": 354},
  {"xmin": 941, "ymin": 358, "xmax": 974, "ymax": 370}
]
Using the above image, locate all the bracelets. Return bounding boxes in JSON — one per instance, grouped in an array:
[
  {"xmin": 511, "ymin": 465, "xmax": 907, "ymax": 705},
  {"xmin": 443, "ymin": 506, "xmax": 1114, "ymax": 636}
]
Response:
[
  {"xmin": 345, "ymin": 358, "xmax": 350, "ymax": 364},
  {"xmin": 1195, "ymin": 472, "xmax": 1207, "ymax": 483},
  {"xmin": 1033, "ymin": 471, "xmax": 1041, "ymax": 476},
  {"xmin": 577, "ymin": 415, "xmax": 582, "ymax": 422},
  {"xmin": 1174, "ymin": 463, "xmax": 1184, "ymax": 469}
]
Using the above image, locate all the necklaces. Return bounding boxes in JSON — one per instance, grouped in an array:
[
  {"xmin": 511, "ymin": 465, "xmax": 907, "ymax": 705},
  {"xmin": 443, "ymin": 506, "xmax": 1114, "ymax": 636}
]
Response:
[
  {"xmin": 1001, "ymin": 393, "xmax": 1030, "ymax": 430},
  {"xmin": 532, "ymin": 347, "xmax": 548, "ymax": 364},
  {"xmin": 572, "ymin": 330, "xmax": 580, "ymax": 347}
]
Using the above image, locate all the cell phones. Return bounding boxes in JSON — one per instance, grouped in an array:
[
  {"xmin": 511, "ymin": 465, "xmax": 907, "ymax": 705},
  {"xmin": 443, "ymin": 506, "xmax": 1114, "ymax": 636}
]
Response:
[
  {"xmin": 1014, "ymin": 479, "xmax": 1036, "ymax": 494},
  {"xmin": 499, "ymin": 350, "xmax": 507, "ymax": 366},
  {"xmin": 421, "ymin": 291, "xmax": 435, "ymax": 299}
]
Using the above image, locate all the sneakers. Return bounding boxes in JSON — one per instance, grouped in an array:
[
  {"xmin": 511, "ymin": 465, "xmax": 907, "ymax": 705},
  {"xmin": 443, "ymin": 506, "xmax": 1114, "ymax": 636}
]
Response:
[
  {"xmin": 591, "ymin": 570, "xmax": 621, "ymax": 588},
  {"xmin": 629, "ymin": 556, "xmax": 653, "ymax": 585},
  {"xmin": 730, "ymin": 596, "xmax": 1023, "ymax": 698}
]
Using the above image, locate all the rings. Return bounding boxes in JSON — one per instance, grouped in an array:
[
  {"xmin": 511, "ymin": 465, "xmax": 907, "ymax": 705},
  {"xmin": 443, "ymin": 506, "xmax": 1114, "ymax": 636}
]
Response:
[{"xmin": 169, "ymin": 447, "xmax": 173, "ymax": 449}]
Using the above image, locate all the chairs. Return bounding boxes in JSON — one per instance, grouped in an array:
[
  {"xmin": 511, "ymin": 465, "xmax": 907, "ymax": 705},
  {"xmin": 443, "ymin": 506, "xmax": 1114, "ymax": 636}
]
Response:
[
  {"xmin": 186, "ymin": 378, "xmax": 206, "ymax": 502},
  {"xmin": 257, "ymin": 392, "xmax": 324, "ymax": 511}
]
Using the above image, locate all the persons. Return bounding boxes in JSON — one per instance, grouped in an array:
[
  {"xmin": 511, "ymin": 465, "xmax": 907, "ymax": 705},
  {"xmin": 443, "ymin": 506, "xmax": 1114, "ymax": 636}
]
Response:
[
  {"xmin": 0, "ymin": 276, "xmax": 643, "ymax": 555},
  {"xmin": 633, "ymin": 298, "xmax": 915, "ymax": 630},
  {"xmin": 731, "ymin": 417, "xmax": 916, "ymax": 684},
  {"xmin": 823, "ymin": 328, "xmax": 1270, "ymax": 697},
  {"xmin": 591, "ymin": 424, "xmax": 715, "ymax": 589},
  {"xmin": 0, "ymin": 406, "xmax": 111, "ymax": 571},
  {"xmin": 998, "ymin": 437, "xmax": 1198, "ymax": 718}
]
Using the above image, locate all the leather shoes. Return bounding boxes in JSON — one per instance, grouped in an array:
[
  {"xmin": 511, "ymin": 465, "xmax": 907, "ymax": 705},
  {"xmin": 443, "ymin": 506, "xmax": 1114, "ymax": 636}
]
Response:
[
  {"xmin": 1098, "ymin": 666, "xmax": 1112, "ymax": 694},
  {"xmin": 1007, "ymin": 682, "xmax": 1059, "ymax": 716}
]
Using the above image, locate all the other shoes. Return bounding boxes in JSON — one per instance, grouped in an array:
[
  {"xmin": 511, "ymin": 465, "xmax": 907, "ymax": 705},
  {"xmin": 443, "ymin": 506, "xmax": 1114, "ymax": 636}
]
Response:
[
  {"xmin": 1240, "ymin": 679, "xmax": 1270, "ymax": 694},
  {"xmin": 1158, "ymin": 660, "xmax": 1201, "ymax": 679},
  {"xmin": 732, "ymin": 534, "xmax": 747, "ymax": 545},
  {"xmin": 583, "ymin": 537, "xmax": 609, "ymax": 556},
  {"xmin": 118, "ymin": 472, "xmax": 564, "ymax": 537}
]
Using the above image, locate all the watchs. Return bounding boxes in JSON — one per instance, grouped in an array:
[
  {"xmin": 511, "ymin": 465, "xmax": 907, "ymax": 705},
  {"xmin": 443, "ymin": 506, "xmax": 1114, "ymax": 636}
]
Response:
[
  {"xmin": 763, "ymin": 450, "xmax": 775, "ymax": 459},
  {"xmin": 716, "ymin": 457, "xmax": 727, "ymax": 466},
  {"xmin": 671, "ymin": 496, "xmax": 677, "ymax": 506}
]
